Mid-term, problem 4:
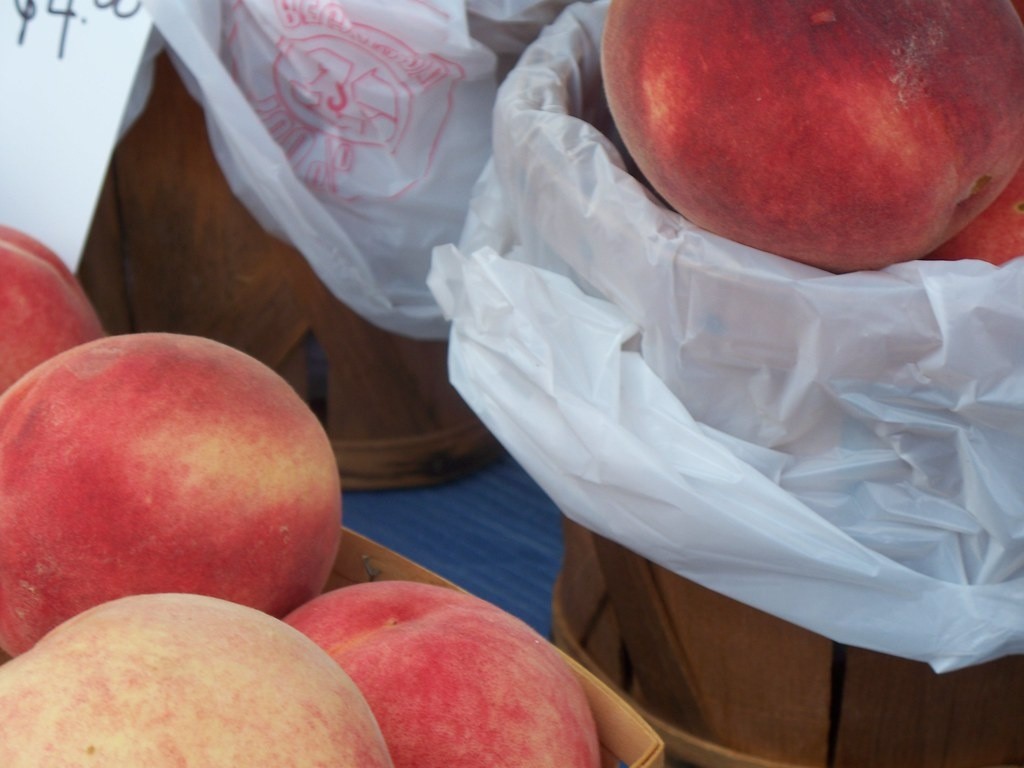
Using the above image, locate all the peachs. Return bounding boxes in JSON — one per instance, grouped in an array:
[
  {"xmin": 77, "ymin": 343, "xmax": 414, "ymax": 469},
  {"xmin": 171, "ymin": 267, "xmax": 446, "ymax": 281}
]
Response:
[
  {"xmin": 0, "ymin": 224, "xmax": 605, "ymax": 768},
  {"xmin": 602, "ymin": 0, "xmax": 1024, "ymax": 275}
]
